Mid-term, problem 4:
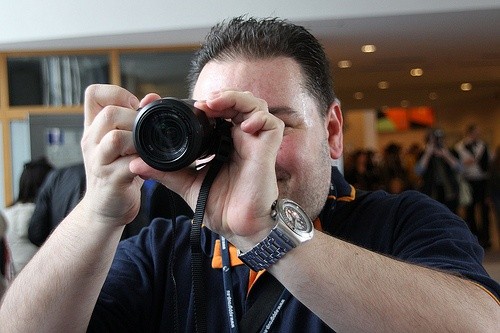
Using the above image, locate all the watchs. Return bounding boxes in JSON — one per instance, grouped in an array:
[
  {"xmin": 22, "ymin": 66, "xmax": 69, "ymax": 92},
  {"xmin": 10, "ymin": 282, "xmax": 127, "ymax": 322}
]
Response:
[{"xmin": 237, "ymin": 198, "xmax": 314, "ymax": 273}]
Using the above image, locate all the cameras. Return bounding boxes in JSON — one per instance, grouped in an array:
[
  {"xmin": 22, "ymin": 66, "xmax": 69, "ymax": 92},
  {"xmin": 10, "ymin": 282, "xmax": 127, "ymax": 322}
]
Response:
[{"xmin": 132, "ymin": 97, "xmax": 235, "ymax": 172}]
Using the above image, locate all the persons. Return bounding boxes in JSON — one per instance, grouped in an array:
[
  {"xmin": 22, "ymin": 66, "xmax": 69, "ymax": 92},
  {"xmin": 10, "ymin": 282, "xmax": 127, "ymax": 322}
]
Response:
[
  {"xmin": 1, "ymin": 14, "xmax": 500, "ymax": 333},
  {"xmin": 27, "ymin": 164, "xmax": 86, "ymax": 247},
  {"xmin": 344, "ymin": 123, "xmax": 500, "ymax": 258},
  {"xmin": 0, "ymin": 156, "xmax": 57, "ymax": 276}
]
